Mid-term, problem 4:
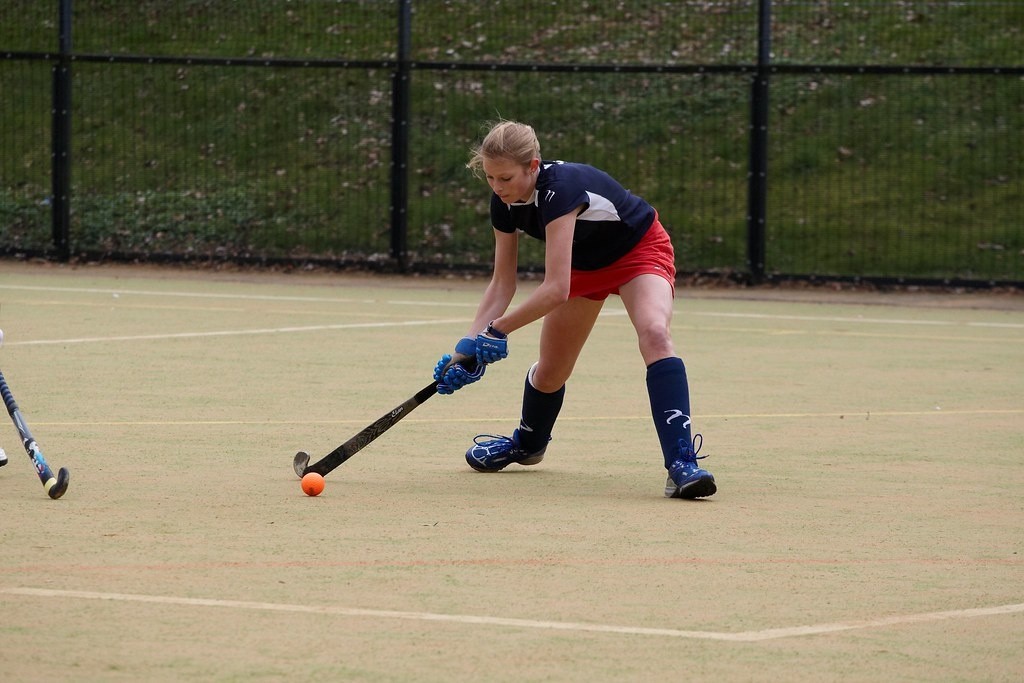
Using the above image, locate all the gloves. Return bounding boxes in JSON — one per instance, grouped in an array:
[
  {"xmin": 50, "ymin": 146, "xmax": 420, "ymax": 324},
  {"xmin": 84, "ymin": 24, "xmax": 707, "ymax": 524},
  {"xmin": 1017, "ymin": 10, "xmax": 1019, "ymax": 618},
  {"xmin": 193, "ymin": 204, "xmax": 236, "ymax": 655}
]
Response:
[
  {"xmin": 433, "ymin": 337, "xmax": 486, "ymax": 394},
  {"xmin": 476, "ymin": 321, "xmax": 509, "ymax": 366}
]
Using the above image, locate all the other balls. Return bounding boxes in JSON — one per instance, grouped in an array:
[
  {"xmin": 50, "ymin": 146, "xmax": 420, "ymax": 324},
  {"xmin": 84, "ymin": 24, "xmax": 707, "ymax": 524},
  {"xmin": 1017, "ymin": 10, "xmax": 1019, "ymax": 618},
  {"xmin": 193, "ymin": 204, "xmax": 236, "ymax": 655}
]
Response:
[{"xmin": 301, "ymin": 472, "xmax": 326, "ymax": 496}]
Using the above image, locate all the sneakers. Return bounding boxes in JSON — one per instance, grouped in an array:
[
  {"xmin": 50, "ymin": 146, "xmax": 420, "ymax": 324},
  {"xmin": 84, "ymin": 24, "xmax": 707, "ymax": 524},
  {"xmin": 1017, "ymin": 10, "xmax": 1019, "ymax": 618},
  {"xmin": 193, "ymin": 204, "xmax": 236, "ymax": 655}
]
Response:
[
  {"xmin": 664, "ymin": 433, "xmax": 717, "ymax": 498},
  {"xmin": 466, "ymin": 429, "xmax": 552, "ymax": 473}
]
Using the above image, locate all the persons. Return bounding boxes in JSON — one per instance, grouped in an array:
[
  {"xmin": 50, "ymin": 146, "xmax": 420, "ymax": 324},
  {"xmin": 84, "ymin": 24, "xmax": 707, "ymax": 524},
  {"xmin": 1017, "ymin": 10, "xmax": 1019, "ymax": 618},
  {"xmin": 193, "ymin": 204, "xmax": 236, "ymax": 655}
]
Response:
[{"xmin": 434, "ymin": 120, "xmax": 718, "ymax": 499}]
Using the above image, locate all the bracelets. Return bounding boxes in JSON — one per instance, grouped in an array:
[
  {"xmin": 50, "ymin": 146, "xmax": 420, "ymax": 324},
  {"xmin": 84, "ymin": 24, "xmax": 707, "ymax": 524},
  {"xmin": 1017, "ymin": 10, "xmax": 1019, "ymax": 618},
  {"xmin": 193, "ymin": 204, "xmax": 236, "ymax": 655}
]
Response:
[{"xmin": 487, "ymin": 320, "xmax": 507, "ymax": 339}]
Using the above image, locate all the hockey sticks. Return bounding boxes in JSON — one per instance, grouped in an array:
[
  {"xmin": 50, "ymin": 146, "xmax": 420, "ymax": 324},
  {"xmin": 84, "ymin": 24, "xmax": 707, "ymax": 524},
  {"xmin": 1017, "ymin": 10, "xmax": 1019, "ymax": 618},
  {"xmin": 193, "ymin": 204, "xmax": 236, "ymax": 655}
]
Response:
[
  {"xmin": 0, "ymin": 364, "xmax": 71, "ymax": 501},
  {"xmin": 293, "ymin": 353, "xmax": 478, "ymax": 479}
]
